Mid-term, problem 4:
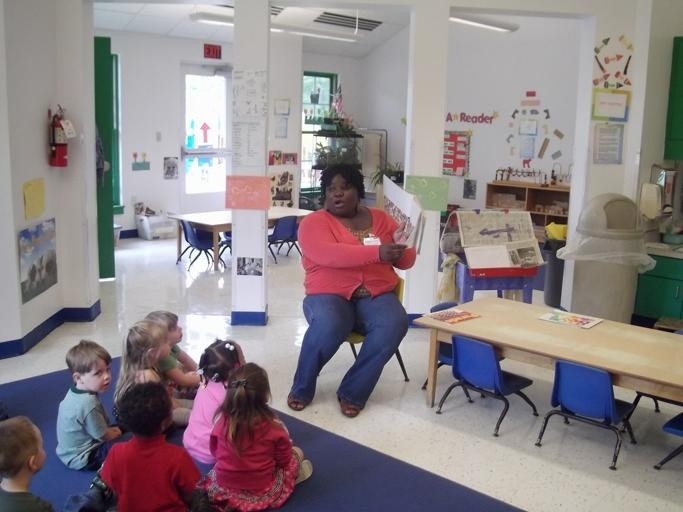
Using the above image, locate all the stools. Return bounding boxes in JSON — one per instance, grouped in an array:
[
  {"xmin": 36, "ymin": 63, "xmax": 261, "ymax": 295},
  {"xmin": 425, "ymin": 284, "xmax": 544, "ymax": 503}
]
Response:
[
  {"xmin": 133, "ymin": 211, "xmax": 179, "ymax": 241},
  {"xmin": 343, "ymin": 331, "xmax": 410, "ymax": 383}
]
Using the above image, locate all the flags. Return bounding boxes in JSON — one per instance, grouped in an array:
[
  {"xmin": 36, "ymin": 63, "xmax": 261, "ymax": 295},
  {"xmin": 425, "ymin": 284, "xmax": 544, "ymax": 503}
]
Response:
[{"xmin": 329, "ymin": 84, "xmax": 347, "ymax": 117}]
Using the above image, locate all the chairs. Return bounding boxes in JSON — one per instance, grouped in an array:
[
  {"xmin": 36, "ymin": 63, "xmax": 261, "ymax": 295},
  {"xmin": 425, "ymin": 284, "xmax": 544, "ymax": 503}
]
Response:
[
  {"xmin": 163, "ymin": 203, "xmax": 316, "ymax": 273},
  {"xmin": 435, "ymin": 330, "xmax": 538, "ymax": 437},
  {"xmin": 419, "ymin": 298, "xmax": 487, "ymax": 404},
  {"xmin": 535, "ymin": 358, "xmax": 638, "ymax": 477},
  {"xmin": 626, "ymin": 327, "xmax": 682, "ymax": 472}
]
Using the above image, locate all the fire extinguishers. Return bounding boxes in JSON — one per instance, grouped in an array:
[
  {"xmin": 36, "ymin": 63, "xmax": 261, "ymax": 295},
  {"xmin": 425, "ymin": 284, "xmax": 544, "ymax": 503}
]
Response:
[{"xmin": 46, "ymin": 103, "xmax": 70, "ymax": 168}]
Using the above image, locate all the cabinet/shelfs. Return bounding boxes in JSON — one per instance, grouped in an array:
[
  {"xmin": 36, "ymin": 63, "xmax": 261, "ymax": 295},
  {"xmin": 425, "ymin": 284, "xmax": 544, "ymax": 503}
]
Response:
[
  {"xmin": 632, "ymin": 253, "xmax": 682, "ymax": 320},
  {"xmin": 483, "ymin": 180, "xmax": 570, "ymax": 247}
]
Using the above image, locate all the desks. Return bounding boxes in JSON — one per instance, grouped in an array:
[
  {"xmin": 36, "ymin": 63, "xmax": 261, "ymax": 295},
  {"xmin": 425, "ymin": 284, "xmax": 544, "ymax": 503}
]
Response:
[{"xmin": 410, "ymin": 294, "xmax": 683, "ymax": 411}]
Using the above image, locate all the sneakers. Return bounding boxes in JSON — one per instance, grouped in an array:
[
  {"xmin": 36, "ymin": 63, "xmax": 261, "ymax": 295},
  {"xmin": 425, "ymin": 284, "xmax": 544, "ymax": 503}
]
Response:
[{"xmin": 295, "ymin": 459, "xmax": 313, "ymax": 486}]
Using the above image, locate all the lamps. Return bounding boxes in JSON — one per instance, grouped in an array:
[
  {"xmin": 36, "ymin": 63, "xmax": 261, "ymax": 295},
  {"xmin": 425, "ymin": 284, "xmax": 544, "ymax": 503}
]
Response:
[
  {"xmin": 185, "ymin": 3, "xmax": 362, "ymax": 46},
  {"xmin": 448, "ymin": 11, "xmax": 519, "ymax": 34}
]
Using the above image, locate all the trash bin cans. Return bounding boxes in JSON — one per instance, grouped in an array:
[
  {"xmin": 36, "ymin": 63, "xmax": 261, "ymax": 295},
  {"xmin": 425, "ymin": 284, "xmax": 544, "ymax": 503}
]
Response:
[{"xmin": 569, "ymin": 190, "xmax": 648, "ymax": 325}]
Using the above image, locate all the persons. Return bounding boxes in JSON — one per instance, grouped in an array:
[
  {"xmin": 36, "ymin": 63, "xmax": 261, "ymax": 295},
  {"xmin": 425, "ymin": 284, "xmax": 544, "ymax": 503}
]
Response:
[
  {"xmin": 287, "ymin": 164, "xmax": 416, "ymax": 418},
  {"xmin": 97, "ymin": 383, "xmax": 209, "ymax": 511},
  {"xmin": 143, "ymin": 310, "xmax": 199, "ymax": 399},
  {"xmin": 54, "ymin": 340, "xmax": 132, "ymax": 473},
  {"xmin": 0, "ymin": 414, "xmax": 117, "ymax": 510},
  {"xmin": 201, "ymin": 363, "xmax": 314, "ymax": 511},
  {"xmin": 182, "ymin": 338, "xmax": 246, "ymax": 477},
  {"xmin": 111, "ymin": 321, "xmax": 195, "ymax": 436}
]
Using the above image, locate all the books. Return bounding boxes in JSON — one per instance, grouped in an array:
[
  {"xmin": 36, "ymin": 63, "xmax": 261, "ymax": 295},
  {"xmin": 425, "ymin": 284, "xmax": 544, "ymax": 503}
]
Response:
[
  {"xmin": 533, "ymin": 221, "xmax": 546, "ymax": 242},
  {"xmin": 377, "ymin": 173, "xmax": 428, "ymax": 257},
  {"xmin": 488, "ymin": 191, "xmax": 526, "ymax": 210},
  {"xmin": 423, "ymin": 309, "xmax": 480, "ymax": 324},
  {"xmin": 538, "ymin": 309, "xmax": 605, "ymax": 330}
]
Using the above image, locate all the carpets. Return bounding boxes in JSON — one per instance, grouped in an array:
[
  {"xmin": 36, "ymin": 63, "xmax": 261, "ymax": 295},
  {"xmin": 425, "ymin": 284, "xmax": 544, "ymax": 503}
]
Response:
[{"xmin": 0, "ymin": 356, "xmax": 528, "ymax": 512}]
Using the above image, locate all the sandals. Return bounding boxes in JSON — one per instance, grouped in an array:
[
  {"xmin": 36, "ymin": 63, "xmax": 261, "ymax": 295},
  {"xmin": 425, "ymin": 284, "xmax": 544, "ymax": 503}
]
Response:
[
  {"xmin": 287, "ymin": 392, "xmax": 307, "ymax": 411},
  {"xmin": 338, "ymin": 395, "xmax": 360, "ymax": 418}
]
